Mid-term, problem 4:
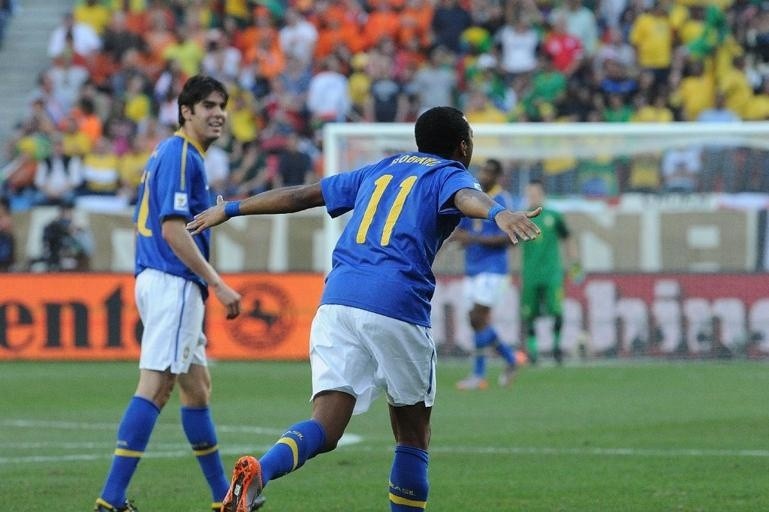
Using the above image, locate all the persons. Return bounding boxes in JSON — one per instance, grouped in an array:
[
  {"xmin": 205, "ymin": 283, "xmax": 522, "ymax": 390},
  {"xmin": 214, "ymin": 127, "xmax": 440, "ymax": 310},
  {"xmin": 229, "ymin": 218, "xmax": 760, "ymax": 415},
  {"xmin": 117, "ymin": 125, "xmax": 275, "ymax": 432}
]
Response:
[
  {"xmin": 90, "ymin": 72, "xmax": 243, "ymax": 512},
  {"xmin": 449, "ymin": 159, "xmax": 526, "ymax": 389},
  {"xmin": 516, "ymin": 183, "xmax": 578, "ymax": 365},
  {"xmin": 185, "ymin": 105, "xmax": 542, "ymax": 510}
]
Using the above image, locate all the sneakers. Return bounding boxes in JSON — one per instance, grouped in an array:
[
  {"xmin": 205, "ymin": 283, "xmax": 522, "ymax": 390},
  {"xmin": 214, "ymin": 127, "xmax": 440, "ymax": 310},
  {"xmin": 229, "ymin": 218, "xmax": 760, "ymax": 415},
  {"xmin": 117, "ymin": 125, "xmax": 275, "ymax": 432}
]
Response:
[
  {"xmin": 93, "ymin": 497, "xmax": 140, "ymax": 512},
  {"xmin": 497, "ymin": 349, "xmax": 529, "ymax": 386},
  {"xmin": 211, "ymin": 454, "xmax": 267, "ymax": 512},
  {"xmin": 454, "ymin": 374, "xmax": 489, "ymax": 392}
]
